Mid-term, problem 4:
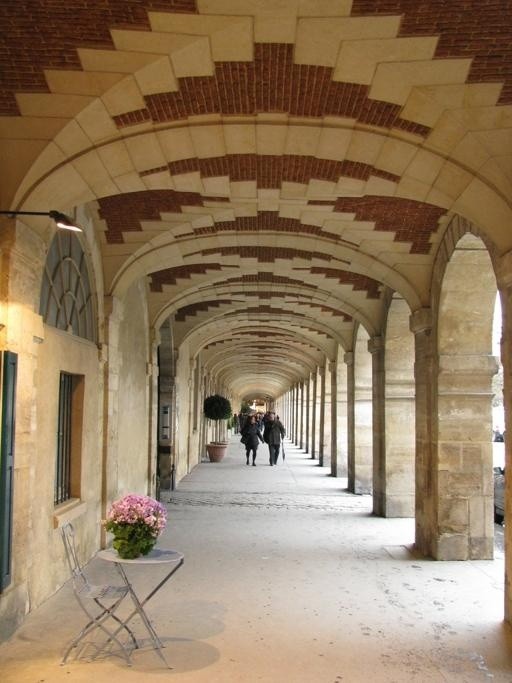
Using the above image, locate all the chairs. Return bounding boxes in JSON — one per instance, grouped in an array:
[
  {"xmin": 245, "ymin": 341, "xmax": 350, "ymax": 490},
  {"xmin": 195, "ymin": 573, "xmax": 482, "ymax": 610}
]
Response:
[{"xmin": 59, "ymin": 520, "xmax": 139, "ymax": 667}]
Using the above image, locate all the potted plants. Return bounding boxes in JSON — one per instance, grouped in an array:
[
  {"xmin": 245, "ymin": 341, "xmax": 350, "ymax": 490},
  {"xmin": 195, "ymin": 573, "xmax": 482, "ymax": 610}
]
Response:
[{"xmin": 203, "ymin": 394, "xmax": 233, "ymax": 462}]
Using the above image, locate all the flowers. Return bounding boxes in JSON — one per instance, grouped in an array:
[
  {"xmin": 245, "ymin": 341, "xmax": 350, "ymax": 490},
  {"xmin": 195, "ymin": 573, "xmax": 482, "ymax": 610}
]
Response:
[{"xmin": 101, "ymin": 494, "xmax": 168, "ymax": 559}]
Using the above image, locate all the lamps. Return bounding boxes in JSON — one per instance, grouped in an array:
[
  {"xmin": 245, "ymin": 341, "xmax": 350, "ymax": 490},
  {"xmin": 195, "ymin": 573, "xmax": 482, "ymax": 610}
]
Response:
[{"xmin": 0, "ymin": 209, "xmax": 85, "ymax": 232}]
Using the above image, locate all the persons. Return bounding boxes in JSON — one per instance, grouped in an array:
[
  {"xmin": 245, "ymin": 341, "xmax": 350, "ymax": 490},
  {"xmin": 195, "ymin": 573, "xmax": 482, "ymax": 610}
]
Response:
[
  {"xmin": 234, "ymin": 412, "xmax": 269, "ymax": 434},
  {"xmin": 263, "ymin": 412, "xmax": 286, "ymax": 466},
  {"xmin": 240, "ymin": 415, "xmax": 264, "ymax": 466}
]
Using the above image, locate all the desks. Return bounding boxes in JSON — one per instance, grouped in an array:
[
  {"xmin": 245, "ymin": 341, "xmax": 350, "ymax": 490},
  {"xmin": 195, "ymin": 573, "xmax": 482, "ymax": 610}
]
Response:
[{"xmin": 86, "ymin": 547, "xmax": 184, "ymax": 669}]
